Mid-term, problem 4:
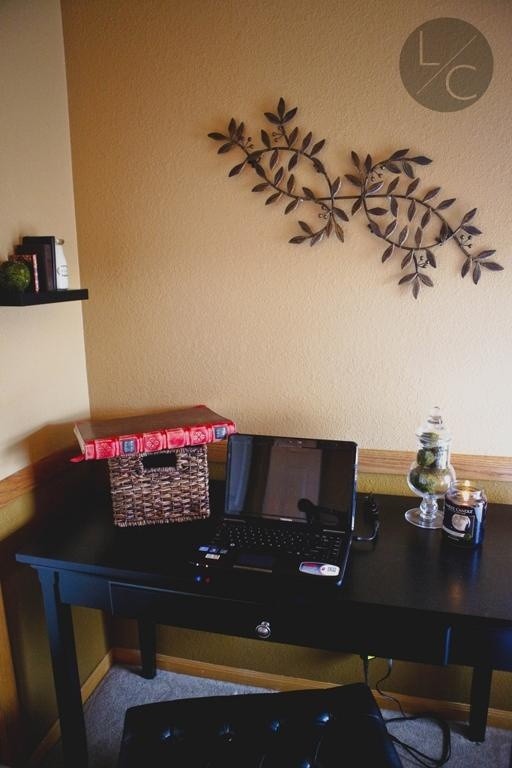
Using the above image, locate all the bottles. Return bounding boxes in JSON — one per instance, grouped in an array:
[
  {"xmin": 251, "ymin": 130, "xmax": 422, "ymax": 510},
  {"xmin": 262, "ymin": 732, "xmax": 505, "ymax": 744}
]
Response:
[
  {"xmin": 56, "ymin": 236, "xmax": 70, "ymax": 291},
  {"xmin": 443, "ymin": 481, "xmax": 488, "ymax": 548}
]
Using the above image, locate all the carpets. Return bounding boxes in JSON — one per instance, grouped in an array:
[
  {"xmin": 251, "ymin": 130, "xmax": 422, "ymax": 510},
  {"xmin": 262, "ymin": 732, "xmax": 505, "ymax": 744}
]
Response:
[{"xmin": 43, "ymin": 664, "xmax": 510, "ymax": 768}]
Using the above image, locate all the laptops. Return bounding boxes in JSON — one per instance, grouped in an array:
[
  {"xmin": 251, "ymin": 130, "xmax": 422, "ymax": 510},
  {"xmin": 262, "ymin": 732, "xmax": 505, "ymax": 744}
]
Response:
[{"xmin": 189, "ymin": 433, "xmax": 359, "ymax": 587}]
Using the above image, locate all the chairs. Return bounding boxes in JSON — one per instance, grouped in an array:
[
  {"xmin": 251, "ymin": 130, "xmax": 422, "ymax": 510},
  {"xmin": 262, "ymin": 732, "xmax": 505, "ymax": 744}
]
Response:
[{"xmin": 119, "ymin": 682, "xmax": 395, "ymax": 765}]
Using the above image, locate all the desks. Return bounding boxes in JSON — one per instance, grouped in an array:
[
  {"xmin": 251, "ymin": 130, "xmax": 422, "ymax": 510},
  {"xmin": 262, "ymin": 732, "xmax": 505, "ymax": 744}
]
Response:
[{"xmin": 1, "ymin": 489, "xmax": 511, "ymax": 768}]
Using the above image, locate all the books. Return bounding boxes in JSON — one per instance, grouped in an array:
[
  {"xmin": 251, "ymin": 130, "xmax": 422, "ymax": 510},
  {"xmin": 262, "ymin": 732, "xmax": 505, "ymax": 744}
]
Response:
[
  {"xmin": 8, "ymin": 234, "xmax": 58, "ymax": 294},
  {"xmin": 69, "ymin": 404, "xmax": 237, "ymax": 461}
]
